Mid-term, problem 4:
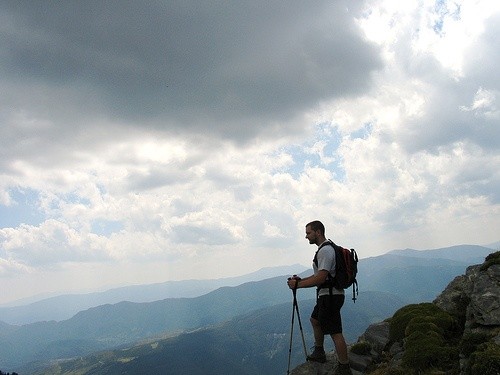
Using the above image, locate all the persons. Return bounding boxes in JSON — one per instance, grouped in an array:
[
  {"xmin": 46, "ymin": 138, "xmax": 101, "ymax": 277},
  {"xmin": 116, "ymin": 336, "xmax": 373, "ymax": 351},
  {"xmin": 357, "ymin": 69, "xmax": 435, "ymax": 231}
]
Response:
[{"xmin": 286, "ymin": 220, "xmax": 359, "ymax": 375}]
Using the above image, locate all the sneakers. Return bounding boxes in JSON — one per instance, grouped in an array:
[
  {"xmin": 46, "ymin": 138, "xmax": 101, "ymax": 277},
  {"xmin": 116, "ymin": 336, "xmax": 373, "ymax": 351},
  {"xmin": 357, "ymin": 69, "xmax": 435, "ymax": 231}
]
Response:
[
  {"xmin": 337, "ymin": 362, "xmax": 352, "ymax": 375},
  {"xmin": 306, "ymin": 346, "xmax": 327, "ymax": 363}
]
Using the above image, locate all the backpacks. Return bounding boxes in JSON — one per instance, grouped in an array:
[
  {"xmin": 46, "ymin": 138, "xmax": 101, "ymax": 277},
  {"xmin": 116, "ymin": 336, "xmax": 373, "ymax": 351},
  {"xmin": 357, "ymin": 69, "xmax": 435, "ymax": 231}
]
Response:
[{"xmin": 313, "ymin": 242, "xmax": 357, "ymax": 291}]
